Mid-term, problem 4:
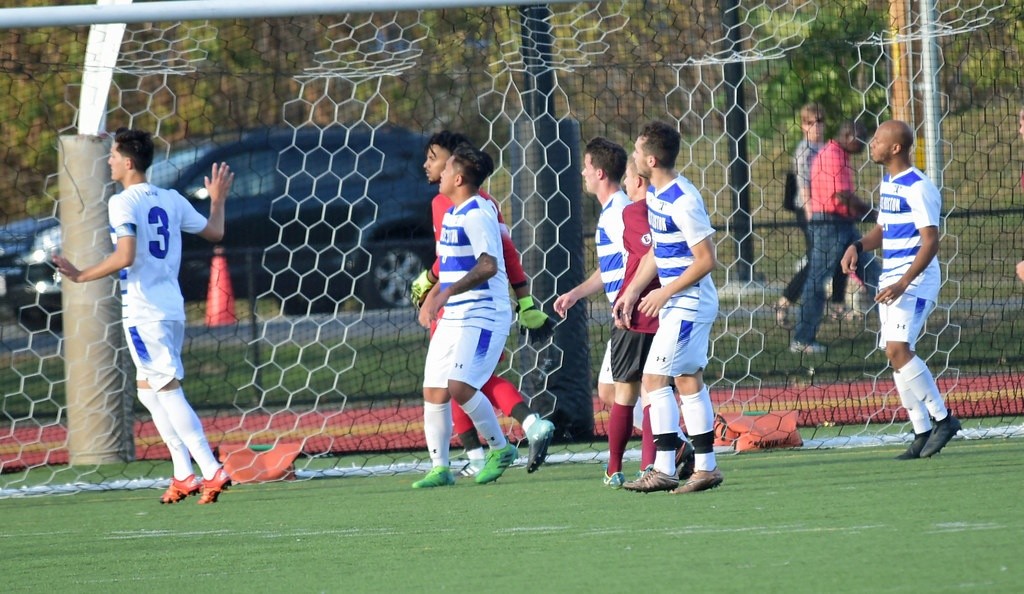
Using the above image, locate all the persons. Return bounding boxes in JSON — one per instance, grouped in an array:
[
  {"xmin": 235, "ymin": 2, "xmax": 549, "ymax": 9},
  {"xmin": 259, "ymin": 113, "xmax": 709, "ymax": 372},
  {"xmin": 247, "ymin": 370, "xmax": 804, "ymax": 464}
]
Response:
[
  {"xmin": 52, "ymin": 127, "xmax": 235, "ymax": 504},
  {"xmin": 555, "ymin": 120, "xmax": 724, "ymax": 494},
  {"xmin": 408, "ymin": 130, "xmax": 554, "ymax": 488},
  {"xmin": 776, "ymin": 103, "xmax": 857, "ymax": 323},
  {"xmin": 791, "ymin": 118, "xmax": 881, "ymax": 353},
  {"xmin": 839, "ymin": 120, "xmax": 961, "ymax": 459}
]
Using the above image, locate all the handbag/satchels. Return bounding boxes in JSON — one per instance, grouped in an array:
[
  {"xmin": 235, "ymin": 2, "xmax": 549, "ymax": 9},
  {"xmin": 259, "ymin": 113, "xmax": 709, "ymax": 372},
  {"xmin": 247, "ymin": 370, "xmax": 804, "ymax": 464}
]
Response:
[{"xmin": 783, "ymin": 173, "xmax": 799, "ymax": 211}]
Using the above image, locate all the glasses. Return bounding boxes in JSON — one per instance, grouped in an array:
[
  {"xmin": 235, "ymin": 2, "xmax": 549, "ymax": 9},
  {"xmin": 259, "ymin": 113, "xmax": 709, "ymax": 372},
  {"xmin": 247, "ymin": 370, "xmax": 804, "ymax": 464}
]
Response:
[{"xmin": 807, "ymin": 118, "xmax": 822, "ymax": 124}]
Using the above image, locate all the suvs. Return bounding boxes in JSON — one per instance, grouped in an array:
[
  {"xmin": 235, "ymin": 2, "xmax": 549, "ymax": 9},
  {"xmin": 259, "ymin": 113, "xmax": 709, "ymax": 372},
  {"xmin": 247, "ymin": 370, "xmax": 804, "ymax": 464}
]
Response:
[{"xmin": 0, "ymin": 120, "xmax": 439, "ymax": 336}]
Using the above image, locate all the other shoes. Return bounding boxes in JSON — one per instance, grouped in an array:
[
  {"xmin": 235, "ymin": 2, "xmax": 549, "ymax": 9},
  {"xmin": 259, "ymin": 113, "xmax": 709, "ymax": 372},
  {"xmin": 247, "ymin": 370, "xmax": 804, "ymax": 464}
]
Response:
[
  {"xmin": 790, "ymin": 340, "xmax": 828, "ymax": 353},
  {"xmin": 773, "ymin": 302, "xmax": 790, "ymax": 331}
]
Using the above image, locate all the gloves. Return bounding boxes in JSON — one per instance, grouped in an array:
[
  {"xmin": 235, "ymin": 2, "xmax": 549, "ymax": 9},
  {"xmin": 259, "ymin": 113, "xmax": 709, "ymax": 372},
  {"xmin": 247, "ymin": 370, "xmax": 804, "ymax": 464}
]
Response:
[
  {"xmin": 410, "ymin": 269, "xmax": 434, "ymax": 310},
  {"xmin": 516, "ymin": 295, "xmax": 557, "ymax": 345}
]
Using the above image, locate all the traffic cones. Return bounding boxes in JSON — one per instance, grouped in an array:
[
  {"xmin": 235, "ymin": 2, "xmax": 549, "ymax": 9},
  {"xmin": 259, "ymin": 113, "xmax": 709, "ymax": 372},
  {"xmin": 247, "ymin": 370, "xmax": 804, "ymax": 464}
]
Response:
[{"xmin": 202, "ymin": 243, "xmax": 237, "ymax": 329}]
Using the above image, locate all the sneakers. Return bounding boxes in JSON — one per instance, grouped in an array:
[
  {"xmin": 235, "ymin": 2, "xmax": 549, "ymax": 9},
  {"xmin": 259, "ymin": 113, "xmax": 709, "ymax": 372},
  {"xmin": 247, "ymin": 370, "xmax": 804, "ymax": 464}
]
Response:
[
  {"xmin": 636, "ymin": 465, "xmax": 653, "ymax": 478},
  {"xmin": 895, "ymin": 430, "xmax": 931, "ymax": 459},
  {"xmin": 604, "ymin": 469, "xmax": 625, "ymax": 490},
  {"xmin": 412, "ymin": 471, "xmax": 455, "ymax": 488},
  {"xmin": 920, "ymin": 409, "xmax": 962, "ymax": 458},
  {"xmin": 160, "ymin": 474, "xmax": 200, "ymax": 504},
  {"xmin": 197, "ymin": 465, "xmax": 232, "ymax": 504},
  {"xmin": 622, "ymin": 468, "xmax": 679, "ymax": 494},
  {"xmin": 475, "ymin": 439, "xmax": 519, "ymax": 484},
  {"xmin": 670, "ymin": 466, "xmax": 724, "ymax": 493},
  {"xmin": 453, "ymin": 460, "xmax": 484, "ymax": 478},
  {"xmin": 526, "ymin": 413, "xmax": 555, "ymax": 473}
]
